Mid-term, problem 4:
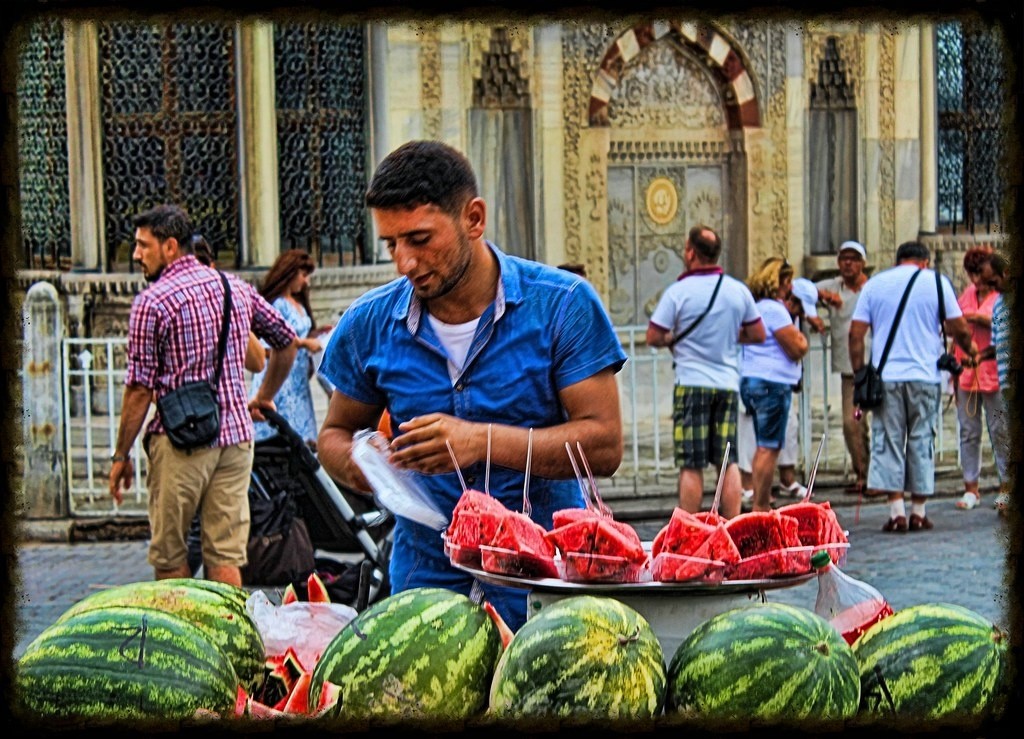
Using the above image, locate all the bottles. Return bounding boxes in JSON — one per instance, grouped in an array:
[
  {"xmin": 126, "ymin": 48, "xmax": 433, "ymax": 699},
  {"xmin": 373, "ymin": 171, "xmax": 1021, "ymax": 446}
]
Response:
[{"xmin": 810, "ymin": 551, "xmax": 893, "ymax": 648}]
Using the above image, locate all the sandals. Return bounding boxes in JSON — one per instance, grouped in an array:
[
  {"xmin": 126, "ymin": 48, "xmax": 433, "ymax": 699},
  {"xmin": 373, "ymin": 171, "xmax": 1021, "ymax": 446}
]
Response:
[
  {"xmin": 741, "ymin": 488, "xmax": 753, "ymax": 506},
  {"xmin": 779, "ymin": 481, "xmax": 816, "ymax": 499}
]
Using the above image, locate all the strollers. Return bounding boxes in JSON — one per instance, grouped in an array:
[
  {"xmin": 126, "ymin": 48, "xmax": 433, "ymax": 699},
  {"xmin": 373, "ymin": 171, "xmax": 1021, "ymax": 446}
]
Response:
[{"xmin": 245, "ymin": 404, "xmax": 396, "ymax": 611}]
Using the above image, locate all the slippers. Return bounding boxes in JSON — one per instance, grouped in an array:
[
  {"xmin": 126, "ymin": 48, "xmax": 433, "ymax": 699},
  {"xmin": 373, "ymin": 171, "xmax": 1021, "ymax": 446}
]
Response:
[
  {"xmin": 954, "ymin": 492, "xmax": 980, "ymax": 509},
  {"xmin": 993, "ymin": 492, "xmax": 1010, "ymax": 508}
]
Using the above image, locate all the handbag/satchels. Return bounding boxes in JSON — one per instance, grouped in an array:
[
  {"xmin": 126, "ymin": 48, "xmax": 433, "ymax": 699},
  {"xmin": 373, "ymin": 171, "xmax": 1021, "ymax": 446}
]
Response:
[
  {"xmin": 852, "ymin": 364, "xmax": 885, "ymax": 411},
  {"xmin": 239, "ymin": 488, "xmax": 316, "ymax": 588},
  {"xmin": 156, "ymin": 380, "xmax": 220, "ymax": 449}
]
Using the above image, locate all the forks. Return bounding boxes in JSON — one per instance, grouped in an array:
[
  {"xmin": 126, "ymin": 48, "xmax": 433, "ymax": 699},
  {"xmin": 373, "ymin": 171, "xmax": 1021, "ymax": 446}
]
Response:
[{"xmin": 439, "ymin": 422, "xmax": 828, "ymax": 526}]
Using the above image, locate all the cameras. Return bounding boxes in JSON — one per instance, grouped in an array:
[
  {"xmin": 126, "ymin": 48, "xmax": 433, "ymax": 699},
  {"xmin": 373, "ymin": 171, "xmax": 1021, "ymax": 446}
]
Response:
[{"xmin": 937, "ymin": 354, "xmax": 964, "ymax": 376}]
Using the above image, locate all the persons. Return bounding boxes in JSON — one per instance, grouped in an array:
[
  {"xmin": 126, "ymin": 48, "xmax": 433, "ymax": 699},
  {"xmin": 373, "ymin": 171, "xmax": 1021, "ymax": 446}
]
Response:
[
  {"xmin": 848, "ymin": 241, "xmax": 979, "ymax": 534},
  {"xmin": 247, "ymin": 248, "xmax": 336, "ymax": 443},
  {"xmin": 943, "ymin": 249, "xmax": 1013, "ymax": 512},
  {"xmin": 645, "ymin": 225, "xmax": 766, "ymax": 520},
  {"xmin": 812, "ymin": 241, "xmax": 889, "ymax": 498},
  {"xmin": 316, "ymin": 137, "xmax": 629, "ymax": 636},
  {"xmin": 736, "ymin": 255, "xmax": 827, "ymax": 513},
  {"xmin": 108, "ymin": 208, "xmax": 300, "ymax": 589}
]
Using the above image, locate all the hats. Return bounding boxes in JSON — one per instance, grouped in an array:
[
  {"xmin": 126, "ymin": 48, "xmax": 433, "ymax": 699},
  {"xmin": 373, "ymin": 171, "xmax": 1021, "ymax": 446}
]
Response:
[
  {"xmin": 791, "ymin": 278, "xmax": 819, "ymax": 317},
  {"xmin": 839, "ymin": 240, "xmax": 866, "ymax": 262}
]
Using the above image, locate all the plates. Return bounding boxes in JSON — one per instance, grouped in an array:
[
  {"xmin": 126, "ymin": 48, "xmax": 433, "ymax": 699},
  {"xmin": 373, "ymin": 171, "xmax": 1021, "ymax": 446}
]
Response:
[{"xmin": 450, "ymin": 541, "xmax": 822, "ymax": 597}]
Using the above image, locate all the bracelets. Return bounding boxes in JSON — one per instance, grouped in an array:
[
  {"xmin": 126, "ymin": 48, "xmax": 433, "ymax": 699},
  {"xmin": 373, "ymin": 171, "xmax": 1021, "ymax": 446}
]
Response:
[{"xmin": 111, "ymin": 453, "xmax": 130, "ymax": 464}]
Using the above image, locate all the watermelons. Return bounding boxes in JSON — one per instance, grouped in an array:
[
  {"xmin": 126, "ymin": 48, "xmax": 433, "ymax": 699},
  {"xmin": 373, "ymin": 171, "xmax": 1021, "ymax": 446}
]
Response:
[
  {"xmin": 444, "ymin": 490, "xmax": 846, "ymax": 583},
  {"xmin": 15, "ymin": 573, "xmax": 1009, "ymax": 721}
]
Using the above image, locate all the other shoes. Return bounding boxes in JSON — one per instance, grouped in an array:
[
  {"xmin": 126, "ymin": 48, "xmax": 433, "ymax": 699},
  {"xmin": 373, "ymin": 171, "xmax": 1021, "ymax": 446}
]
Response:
[
  {"xmin": 864, "ymin": 488, "xmax": 883, "ymax": 496},
  {"xmin": 844, "ymin": 483, "xmax": 867, "ymax": 494},
  {"xmin": 908, "ymin": 513, "xmax": 934, "ymax": 530},
  {"xmin": 883, "ymin": 516, "xmax": 907, "ymax": 532}
]
